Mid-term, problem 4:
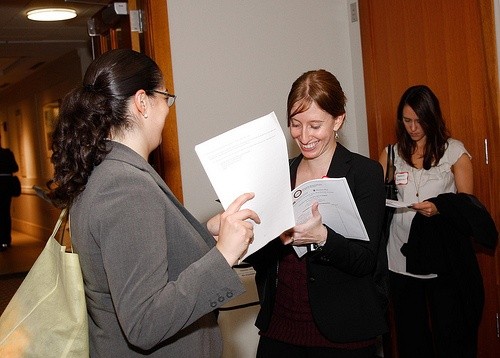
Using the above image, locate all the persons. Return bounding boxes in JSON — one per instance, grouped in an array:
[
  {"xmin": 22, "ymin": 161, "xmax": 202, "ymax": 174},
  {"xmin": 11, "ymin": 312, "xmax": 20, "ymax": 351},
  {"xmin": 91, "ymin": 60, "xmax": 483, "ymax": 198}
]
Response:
[
  {"xmin": 376, "ymin": 83, "xmax": 474, "ymax": 358},
  {"xmin": 215, "ymin": 70, "xmax": 388, "ymax": 358},
  {"xmin": 0, "ymin": 146, "xmax": 21, "ymax": 250},
  {"xmin": 32, "ymin": 49, "xmax": 262, "ymax": 357}
]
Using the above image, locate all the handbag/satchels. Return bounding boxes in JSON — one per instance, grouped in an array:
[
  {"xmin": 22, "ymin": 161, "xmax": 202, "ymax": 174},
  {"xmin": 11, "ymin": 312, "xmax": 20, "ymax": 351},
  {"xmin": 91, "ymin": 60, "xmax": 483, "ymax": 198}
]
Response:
[
  {"xmin": 381, "ymin": 144, "xmax": 398, "ymax": 247},
  {"xmin": 0, "ymin": 205, "xmax": 90, "ymax": 358}
]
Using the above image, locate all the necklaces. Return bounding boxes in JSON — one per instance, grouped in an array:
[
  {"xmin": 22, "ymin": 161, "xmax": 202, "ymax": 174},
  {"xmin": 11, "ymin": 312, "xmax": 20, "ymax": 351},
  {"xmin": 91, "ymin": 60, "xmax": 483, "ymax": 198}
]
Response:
[{"xmin": 411, "ymin": 167, "xmax": 424, "ymax": 198}]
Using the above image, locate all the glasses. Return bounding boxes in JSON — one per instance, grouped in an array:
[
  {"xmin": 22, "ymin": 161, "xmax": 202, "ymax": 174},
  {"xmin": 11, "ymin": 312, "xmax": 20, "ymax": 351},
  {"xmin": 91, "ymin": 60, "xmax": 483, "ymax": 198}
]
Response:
[{"xmin": 145, "ymin": 89, "xmax": 177, "ymax": 108}]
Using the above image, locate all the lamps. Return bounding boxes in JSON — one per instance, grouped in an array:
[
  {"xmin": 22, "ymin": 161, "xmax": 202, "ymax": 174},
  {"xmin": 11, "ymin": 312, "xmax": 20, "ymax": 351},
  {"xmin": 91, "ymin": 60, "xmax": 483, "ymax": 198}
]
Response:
[{"xmin": 23, "ymin": 8, "xmax": 81, "ymax": 22}]
